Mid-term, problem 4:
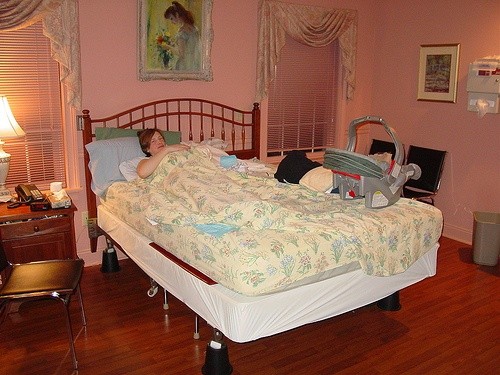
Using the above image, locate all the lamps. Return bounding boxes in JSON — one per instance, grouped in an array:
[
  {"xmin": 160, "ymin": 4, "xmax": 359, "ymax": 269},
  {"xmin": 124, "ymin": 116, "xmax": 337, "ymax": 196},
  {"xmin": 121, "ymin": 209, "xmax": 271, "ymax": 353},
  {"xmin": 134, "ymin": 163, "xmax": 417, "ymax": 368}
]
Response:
[{"xmin": 0, "ymin": 97, "xmax": 27, "ymax": 202}]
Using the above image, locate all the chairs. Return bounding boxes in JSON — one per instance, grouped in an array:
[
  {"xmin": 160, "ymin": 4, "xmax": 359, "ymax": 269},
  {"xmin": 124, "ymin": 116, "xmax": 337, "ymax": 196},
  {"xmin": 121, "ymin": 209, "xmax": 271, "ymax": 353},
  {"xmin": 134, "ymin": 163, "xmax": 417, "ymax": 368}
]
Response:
[
  {"xmin": 369, "ymin": 138, "xmax": 406, "ymax": 163},
  {"xmin": 0, "ymin": 237, "xmax": 87, "ymax": 375},
  {"xmin": 400, "ymin": 145, "xmax": 448, "ymax": 207}
]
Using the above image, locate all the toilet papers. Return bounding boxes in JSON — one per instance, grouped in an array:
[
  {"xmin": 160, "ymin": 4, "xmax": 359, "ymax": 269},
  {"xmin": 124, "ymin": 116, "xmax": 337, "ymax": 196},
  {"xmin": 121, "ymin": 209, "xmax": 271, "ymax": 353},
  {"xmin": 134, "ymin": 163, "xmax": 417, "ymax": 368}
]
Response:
[{"xmin": 50, "ymin": 182, "xmax": 63, "ymax": 191}]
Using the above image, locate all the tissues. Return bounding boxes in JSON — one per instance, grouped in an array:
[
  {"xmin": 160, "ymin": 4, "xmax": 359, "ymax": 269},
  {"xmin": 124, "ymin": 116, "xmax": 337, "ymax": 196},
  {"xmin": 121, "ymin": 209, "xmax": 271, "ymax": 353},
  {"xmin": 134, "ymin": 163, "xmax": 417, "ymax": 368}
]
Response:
[{"xmin": 48, "ymin": 192, "xmax": 72, "ymax": 208}]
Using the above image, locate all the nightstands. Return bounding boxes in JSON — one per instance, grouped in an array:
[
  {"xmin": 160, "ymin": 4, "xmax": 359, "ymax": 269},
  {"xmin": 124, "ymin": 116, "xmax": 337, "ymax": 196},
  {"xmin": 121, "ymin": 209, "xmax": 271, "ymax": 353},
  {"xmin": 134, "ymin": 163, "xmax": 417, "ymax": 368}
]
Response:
[{"xmin": 0, "ymin": 184, "xmax": 78, "ymax": 313}]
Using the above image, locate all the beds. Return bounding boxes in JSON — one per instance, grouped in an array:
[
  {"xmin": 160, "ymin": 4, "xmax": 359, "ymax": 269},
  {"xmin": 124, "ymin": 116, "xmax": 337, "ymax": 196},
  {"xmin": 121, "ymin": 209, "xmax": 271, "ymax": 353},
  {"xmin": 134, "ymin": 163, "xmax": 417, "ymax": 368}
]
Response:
[{"xmin": 77, "ymin": 96, "xmax": 444, "ymax": 375}]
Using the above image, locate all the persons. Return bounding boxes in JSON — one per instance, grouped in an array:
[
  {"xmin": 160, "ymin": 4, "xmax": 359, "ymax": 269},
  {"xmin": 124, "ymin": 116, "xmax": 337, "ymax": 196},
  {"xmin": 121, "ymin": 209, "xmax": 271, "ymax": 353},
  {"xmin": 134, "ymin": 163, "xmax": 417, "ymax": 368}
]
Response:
[{"xmin": 136, "ymin": 127, "xmax": 191, "ymax": 178}]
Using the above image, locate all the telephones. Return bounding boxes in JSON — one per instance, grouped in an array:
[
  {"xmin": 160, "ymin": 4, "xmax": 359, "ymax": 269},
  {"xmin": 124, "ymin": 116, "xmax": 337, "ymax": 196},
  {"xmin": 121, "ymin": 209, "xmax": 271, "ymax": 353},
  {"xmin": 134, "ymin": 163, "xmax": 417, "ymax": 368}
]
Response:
[{"xmin": 14, "ymin": 183, "xmax": 45, "ymax": 203}]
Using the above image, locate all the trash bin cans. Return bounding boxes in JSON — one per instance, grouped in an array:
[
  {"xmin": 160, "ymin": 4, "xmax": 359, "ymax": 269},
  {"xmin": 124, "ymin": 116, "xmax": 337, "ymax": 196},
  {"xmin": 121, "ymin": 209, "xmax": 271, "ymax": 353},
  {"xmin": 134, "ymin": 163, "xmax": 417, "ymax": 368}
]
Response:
[{"xmin": 472, "ymin": 210, "xmax": 500, "ymax": 267}]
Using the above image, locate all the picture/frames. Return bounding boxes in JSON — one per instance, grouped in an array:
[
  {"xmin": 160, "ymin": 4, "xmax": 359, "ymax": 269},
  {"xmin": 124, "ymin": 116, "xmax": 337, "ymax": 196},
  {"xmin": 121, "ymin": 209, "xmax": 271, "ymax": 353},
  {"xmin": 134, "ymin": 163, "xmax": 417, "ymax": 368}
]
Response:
[
  {"xmin": 136, "ymin": 0, "xmax": 215, "ymax": 83},
  {"xmin": 416, "ymin": 42, "xmax": 461, "ymax": 104}
]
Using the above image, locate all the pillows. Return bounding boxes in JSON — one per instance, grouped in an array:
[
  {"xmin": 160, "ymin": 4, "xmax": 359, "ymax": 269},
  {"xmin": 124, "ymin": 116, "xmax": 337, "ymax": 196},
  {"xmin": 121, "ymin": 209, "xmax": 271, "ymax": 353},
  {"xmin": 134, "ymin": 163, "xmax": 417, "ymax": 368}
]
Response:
[
  {"xmin": 93, "ymin": 127, "xmax": 181, "ymax": 145},
  {"xmin": 118, "ymin": 154, "xmax": 151, "ymax": 182},
  {"xmin": 85, "ymin": 137, "xmax": 149, "ymax": 197}
]
